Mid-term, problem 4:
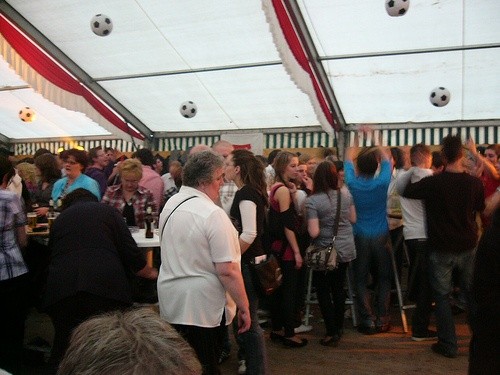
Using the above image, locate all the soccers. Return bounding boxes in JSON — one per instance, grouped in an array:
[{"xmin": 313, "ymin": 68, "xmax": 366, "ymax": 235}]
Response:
[
  {"xmin": 383, "ymin": 0, "xmax": 410, "ymax": 16},
  {"xmin": 179, "ymin": 100, "xmax": 197, "ymax": 116},
  {"xmin": 20, "ymin": 108, "xmax": 34, "ymax": 121},
  {"xmin": 429, "ymin": 86, "xmax": 450, "ymax": 106},
  {"xmin": 89, "ymin": 15, "xmax": 113, "ymax": 38}
]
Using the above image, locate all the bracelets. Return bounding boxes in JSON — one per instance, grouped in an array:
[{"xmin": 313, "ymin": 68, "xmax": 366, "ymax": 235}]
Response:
[{"xmin": 476, "ymin": 151, "xmax": 480, "ymax": 158}]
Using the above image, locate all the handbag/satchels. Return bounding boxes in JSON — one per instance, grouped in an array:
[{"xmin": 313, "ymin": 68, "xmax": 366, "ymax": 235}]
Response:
[
  {"xmin": 254, "ymin": 254, "xmax": 282, "ymax": 292},
  {"xmin": 304, "ymin": 243, "xmax": 337, "ymax": 271}
]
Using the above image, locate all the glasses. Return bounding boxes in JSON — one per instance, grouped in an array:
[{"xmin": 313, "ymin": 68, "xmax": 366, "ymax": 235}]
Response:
[{"xmin": 62, "ymin": 159, "xmax": 79, "ymax": 166}]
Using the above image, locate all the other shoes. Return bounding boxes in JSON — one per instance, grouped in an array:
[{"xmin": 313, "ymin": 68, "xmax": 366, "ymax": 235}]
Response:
[
  {"xmin": 439, "ymin": 341, "xmax": 458, "ymax": 357},
  {"xmin": 217, "ymin": 351, "xmax": 228, "ymax": 364},
  {"xmin": 412, "ymin": 328, "xmax": 438, "ymax": 341},
  {"xmin": 359, "ymin": 322, "xmax": 375, "ymax": 334},
  {"xmin": 295, "ymin": 324, "xmax": 313, "ymax": 334},
  {"xmin": 376, "ymin": 323, "xmax": 390, "ymax": 333},
  {"xmin": 238, "ymin": 360, "xmax": 247, "ymax": 375},
  {"xmin": 320, "ymin": 335, "xmax": 336, "ymax": 345},
  {"xmin": 258, "ymin": 319, "xmax": 272, "ymax": 329}
]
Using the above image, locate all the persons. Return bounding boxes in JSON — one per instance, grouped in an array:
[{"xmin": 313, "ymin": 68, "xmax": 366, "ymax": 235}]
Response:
[{"xmin": 0, "ymin": 135, "xmax": 500, "ymax": 375}]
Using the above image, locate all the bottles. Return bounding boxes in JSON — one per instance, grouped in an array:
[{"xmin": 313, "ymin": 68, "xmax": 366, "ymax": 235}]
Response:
[{"xmin": 144, "ymin": 205, "xmax": 155, "ymax": 238}]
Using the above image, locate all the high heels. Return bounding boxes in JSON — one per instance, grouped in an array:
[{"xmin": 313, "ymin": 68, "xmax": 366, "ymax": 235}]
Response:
[
  {"xmin": 270, "ymin": 331, "xmax": 285, "ymax": 342},
  {"xmin": 284, "ymin": 337, "xmax": 307, "ymax": 348}
]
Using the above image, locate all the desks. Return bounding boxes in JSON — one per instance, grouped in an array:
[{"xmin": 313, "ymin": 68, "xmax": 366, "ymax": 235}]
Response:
[
  {"xmin": 129, "ymin": 227, "xmax": 161, "ymax": 269},
  {"xmin": 25, "ymin": 221, "xmax": 51, "ymax": 242}
]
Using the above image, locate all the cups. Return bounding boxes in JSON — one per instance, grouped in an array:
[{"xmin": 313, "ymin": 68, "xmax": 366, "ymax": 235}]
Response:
[
  {"xmin": 26, "ymin": 212, "xmax": 37, "ymax": 233},
  {"xmin": 48, "ymin": 212, "xmax": 60, "ymax": 229}
]
